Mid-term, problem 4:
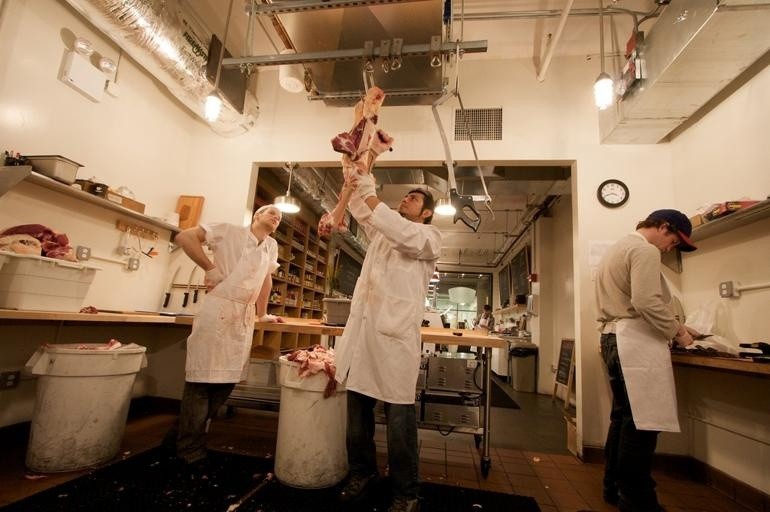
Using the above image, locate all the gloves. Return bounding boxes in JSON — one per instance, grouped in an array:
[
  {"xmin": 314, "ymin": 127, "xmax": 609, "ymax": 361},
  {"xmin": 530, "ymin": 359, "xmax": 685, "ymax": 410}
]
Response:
[
  {"xmin": 260, "ymin": 314, "xmax": 283, "ymax": 324},
  {"xmin": 204, "ymin": 267, "xmax": 224, "ymax": 290},
  {"xmin": 352, "ymin": 167, "xmax": 377, "ymax": 202}
]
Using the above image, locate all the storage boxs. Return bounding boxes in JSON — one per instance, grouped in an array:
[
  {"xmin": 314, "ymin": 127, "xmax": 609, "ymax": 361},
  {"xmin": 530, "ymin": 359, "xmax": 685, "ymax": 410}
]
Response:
[{"xmin": 0, "ymin": 248, "xmax": 104, "ymax": 313}]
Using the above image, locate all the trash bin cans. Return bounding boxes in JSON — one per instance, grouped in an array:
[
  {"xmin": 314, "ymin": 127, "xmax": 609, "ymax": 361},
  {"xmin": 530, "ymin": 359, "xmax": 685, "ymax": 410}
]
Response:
[
  {"xmin": 24, "ymin": 343, "xmax": 148, "ymax": 473},
  {"xmin": 510, "ymin": 342, "xmax": 537, "ymax": 392},
  {"xmin": 274, "ymin": 354, "xmax": 351, "ymax": 489}
]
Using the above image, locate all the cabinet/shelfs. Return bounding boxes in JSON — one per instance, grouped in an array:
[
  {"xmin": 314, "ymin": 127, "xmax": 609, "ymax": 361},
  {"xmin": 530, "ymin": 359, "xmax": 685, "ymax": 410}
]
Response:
[{"xmin": 251, "ymin": 189, "xmax": 334, "ymax": 361}]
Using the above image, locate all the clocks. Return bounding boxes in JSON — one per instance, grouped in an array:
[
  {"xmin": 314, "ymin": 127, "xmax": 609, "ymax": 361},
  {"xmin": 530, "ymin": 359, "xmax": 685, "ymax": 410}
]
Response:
[{"xmin": 598, "ymin": 178, "xmax": 630, "ymax": 209}]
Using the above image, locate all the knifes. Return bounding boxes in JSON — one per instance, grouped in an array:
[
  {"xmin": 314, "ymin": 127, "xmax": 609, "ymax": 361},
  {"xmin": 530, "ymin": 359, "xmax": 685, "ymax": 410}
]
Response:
[
  {"xmin": 182, "ymin": 261, "xmax": 198, "ymax": 308},
  {"xmin": 193, "ymin": 271, "xmax": 202, "ymax": 303},
  {"xmin": 162, "ymin": 265, "xmax": 182, "ymax": 308}
]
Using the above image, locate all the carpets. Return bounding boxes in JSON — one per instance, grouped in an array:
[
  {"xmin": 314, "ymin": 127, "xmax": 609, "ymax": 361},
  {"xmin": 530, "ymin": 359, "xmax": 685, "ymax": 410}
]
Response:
[{"xmin": 0, "ymin": 443, "xmax": 548, "ymax": 512}]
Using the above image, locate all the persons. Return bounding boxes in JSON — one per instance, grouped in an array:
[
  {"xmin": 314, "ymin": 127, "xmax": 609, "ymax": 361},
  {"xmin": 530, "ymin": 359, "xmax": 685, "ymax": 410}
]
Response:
[
  {"xmin": 473, "ymin": 304, "xmax": 494, "ymax": 361},
  {"xmin": 593, "ymin": 207, "xmax": 708, "ymax": 510},
  {"xmin": 153, "ymin": 201, "xmax": 284, "ymax": 475},
  {"xmin": 328, "ymin": 173, "xmax": 445, "ymax": 512}
]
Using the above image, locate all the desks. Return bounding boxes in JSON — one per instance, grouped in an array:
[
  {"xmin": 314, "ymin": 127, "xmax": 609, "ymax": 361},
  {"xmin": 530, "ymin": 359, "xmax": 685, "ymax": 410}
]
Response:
[
  {"xmin": 0, "ymin": 308, "xmax": 508, "ymax": 480},
  {"xmin": 665, "ymin": 350, "xmax": 770, "ymax": 379}
]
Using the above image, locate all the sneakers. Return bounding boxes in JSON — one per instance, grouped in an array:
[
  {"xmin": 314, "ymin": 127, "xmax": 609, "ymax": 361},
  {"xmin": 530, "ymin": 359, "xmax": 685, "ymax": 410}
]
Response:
[
  {"xmin": 343, "ymin": 470, "xmax": 376, "ymax": 500},
  {"xmin": 389, "ymin": 497, "xmax": 420, "ymax": 511},
  {"xmin": 601, "ymin": 487, "xmax": 659, "ymax": 510}
]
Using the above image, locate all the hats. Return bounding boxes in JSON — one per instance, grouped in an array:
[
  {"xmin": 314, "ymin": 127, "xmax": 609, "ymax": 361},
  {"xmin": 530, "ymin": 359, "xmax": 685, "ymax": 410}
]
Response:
[{"xmin": 649, "ymin": 209, "xmax": 698, "ymax": 251}]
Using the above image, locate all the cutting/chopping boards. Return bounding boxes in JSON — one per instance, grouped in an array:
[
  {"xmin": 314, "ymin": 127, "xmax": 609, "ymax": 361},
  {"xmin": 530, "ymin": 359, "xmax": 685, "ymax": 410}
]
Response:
[
  {"xmin": 173, "ymin": 194, "xmax": 204, "ymax": 231},
  {"xmin": 94, "ymin": 307, "xmax": 161, "ymax": 315}
]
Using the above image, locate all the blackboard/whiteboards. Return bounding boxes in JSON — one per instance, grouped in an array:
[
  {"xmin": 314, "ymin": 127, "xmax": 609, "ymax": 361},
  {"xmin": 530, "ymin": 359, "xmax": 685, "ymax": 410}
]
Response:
[
  {"xmin": 498, "ymin": 245, "xmax": 532, "ymax": 310},
  {"xmin": 554, "ymin": 338, "xmax": 575, "ymax": 388}
]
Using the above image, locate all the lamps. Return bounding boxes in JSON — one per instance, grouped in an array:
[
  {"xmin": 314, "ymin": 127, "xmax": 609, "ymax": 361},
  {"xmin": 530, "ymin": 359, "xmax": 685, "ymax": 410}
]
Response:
[
  {"xmin": 202, "ymin": 0, "xmax": 236, "ymax": 124},
  {"xmin": 275, "ymin": 162, "xmax": 303, "ymax": 215},
  {"xmin": 593, "ymin": 0, "xmax": 615, "ymax": 109},
  {"xmin": 57, "ymin": 38, "xmax": 118, "ymax": 104},
  {"xmin": 434, "ymin": 191, "xmax": 458, "ymax": 218}
]
